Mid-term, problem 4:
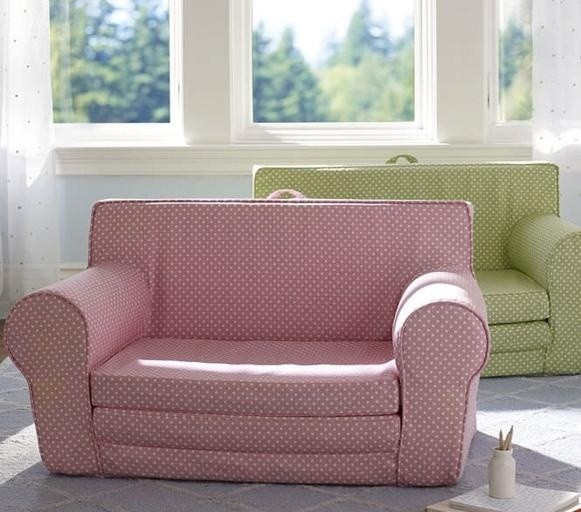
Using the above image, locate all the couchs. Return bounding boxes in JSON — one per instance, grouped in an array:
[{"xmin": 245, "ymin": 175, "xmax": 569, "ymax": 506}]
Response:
[
  {"xmin": 4, "ymin": 190, "xmax": 489, "ymax": 491},
  {"xmin": 252, "ymin": 152, "xmax": 580, "ymax": 378}
]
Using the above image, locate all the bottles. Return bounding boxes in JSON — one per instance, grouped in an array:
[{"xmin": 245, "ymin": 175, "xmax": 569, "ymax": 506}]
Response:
[{"xmin": 486, "ymin": 445, "xmax": 517, "ymax": 501}]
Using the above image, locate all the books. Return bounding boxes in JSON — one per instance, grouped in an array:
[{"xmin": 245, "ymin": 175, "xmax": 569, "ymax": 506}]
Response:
[{"xmin": 448, "ymin": 476, "xmax": 580, "ymax": 512}]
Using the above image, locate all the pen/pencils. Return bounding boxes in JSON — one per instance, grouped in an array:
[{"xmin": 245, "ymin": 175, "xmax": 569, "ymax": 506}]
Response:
[{"xmin": 499, "ymin": 425, "xmax": 513, "ymax": 451}]
